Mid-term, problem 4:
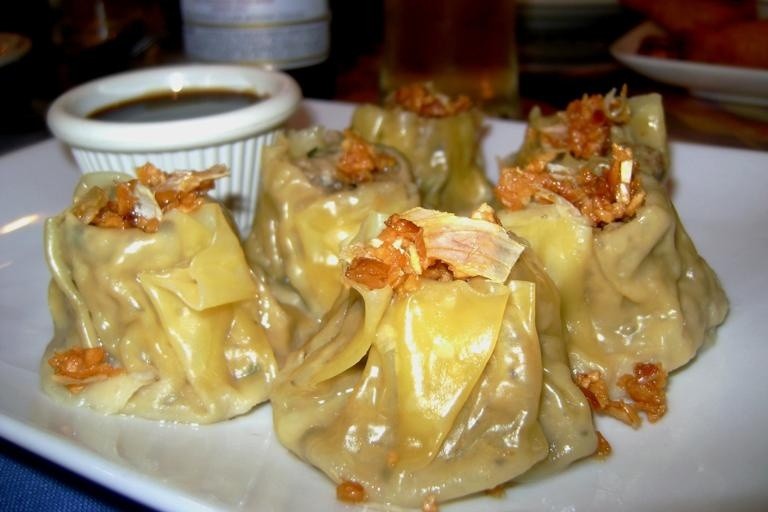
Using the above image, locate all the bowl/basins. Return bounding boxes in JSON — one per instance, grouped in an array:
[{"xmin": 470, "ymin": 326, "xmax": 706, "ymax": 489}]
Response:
[{"xmin": 46, "ymin": 61, "xmax": 301, "ymax": 240}]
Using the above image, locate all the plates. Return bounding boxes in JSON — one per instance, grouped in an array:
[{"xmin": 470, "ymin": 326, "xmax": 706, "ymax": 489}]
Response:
[
  {"xmin": 612, "ymin": 21, "xmax": 767, "ymax": 122},
  {"xmin": 0, "ymin": 89, "xmax": 762, "ymax": 509}
]
